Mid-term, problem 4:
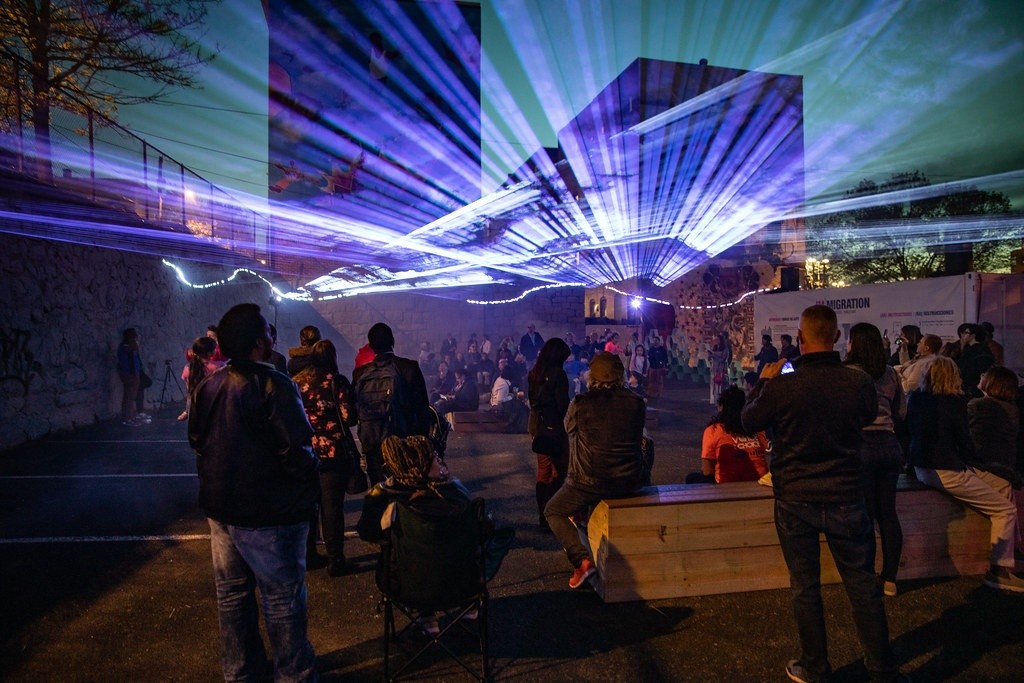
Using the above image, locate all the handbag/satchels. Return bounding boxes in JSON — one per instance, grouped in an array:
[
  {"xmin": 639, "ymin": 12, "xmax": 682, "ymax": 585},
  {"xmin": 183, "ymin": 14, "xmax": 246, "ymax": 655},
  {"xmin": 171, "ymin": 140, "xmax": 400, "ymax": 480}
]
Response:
[
  {"xmin": 713, "ymin": 371, "xmax": 724, "ymax": 384},
  {"xmin": 491, "ymin": 404, "xmax": 504, "ymax": 417}
]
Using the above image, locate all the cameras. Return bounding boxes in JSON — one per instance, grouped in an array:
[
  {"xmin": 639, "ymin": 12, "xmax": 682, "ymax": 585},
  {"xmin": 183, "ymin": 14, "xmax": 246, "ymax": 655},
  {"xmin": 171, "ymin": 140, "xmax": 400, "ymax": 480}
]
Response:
[
  {"xmin": 895, "ymin": 338, "xmax": 903, "ymax": 345},
  {"xmin": 780, "ymin": 361, "xmax": 794, "ymax": 375}
]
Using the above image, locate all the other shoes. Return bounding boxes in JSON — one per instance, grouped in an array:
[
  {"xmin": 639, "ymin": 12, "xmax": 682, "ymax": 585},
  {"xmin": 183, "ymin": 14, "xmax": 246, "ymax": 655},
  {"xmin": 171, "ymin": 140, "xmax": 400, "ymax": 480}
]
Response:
[
  {"xmin": 462, "ymin": 600, "xmax": 483, "ymax": 620},
  {"xmin": 338, "ymin": 462, "xmax": 368, "ymax": 494},
  {"xmin": 875, "ymin": 573, "xmax": 897, "ymax": 596},
  {"xmin": 421, "ymin": 615, "xmax": 440, "ymax": 635},
  {"xmin": 569, "ymin": 556, "xmax": 596, "ymax": 589},
  {"xmin": 121, "ymin": 415, "xmax": 142, "ymax": 427},
  {"xmin": 177, "ymin": 410, "xmax": 188, "ymax": 421},
  {"xmin": 327, "ymin": 558, "xmax": 356, "ymax": 577},
  {"xmin": 786, "ymin": 659, "xmax": 810, "ymax": 683},
  {"xmin": 135, "ymin": 412, "xmax": 152, "ymax": 424},
  {"xmin": 984, "ymin": 568, "xmax": 1024, "ymax": 592}
]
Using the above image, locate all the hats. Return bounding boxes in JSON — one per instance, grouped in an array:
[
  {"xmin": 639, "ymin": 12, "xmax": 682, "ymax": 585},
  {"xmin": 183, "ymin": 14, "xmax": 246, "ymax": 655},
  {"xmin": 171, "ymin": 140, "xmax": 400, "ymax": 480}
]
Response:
[{"xmin": 590, "ymin": 351, "xmax": 624, "ymax": 382}]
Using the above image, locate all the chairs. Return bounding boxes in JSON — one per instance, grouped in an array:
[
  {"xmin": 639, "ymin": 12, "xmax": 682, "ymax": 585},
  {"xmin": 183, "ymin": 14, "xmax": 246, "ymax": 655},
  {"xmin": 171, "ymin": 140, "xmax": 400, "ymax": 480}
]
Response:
[{"xmin": 382, "ymin": 499, "xmax": 515, "ymax": 683}]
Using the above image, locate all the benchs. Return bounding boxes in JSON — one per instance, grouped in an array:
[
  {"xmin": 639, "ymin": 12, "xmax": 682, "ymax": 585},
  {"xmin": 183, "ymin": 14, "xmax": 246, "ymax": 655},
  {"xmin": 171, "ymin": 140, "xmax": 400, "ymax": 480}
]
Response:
[
  {"xmin": 587, "ymin": 484, "xmax": 995, "ymax": 604},
  {"xmin": 449, "ymin": 411, "xmax": 507, "ymax": 435}
]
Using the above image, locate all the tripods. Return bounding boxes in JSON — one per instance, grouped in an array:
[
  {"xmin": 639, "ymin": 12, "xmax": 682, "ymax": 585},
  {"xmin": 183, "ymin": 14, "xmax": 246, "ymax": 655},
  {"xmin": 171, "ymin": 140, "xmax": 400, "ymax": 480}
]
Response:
[{"xmin": 159, "ymin": 366, "xmax": 188, "ymax": 410}]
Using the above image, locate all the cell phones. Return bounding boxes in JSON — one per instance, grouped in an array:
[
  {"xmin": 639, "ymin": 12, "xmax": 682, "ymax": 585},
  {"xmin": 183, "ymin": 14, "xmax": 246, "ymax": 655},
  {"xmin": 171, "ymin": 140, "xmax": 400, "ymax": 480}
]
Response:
[{"xmin": 883, "ymin": 330, "xmax": 888, "ymax": 339}]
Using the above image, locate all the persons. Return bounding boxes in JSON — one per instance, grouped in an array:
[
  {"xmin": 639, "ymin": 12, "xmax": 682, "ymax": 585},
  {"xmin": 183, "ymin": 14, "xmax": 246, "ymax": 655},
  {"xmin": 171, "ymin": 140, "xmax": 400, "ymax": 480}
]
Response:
[
  {"xmin": 544, "ymin": 352, "xmax": 646, "ymax": 588},
  {"xmin": 177, "ymin": 326, "xmax": 377, "ymax": 475},
  {"xmin": 291, "ymin": 338, "xmax": 359, "ymax": 577},
  {"xmin": 833, "ymin": 323, "xmax": 903, "ymax": 595},
  {"xmin": 420, "ymin": 324, "xmax": 670, "ymax": 436},
  {"xmin": 357, "ymin": 436, "xmax": 484, "ymax": 635},
  {"xmin": 188, "ymin": 303, "xmax": 321, "ymax": 683},
  {"xmin": 686, "ymin": 323, "xmax": 1024, "ymax": 494},
  {"xmin": 351, "ymin": 322, "xmax": 431, "ymax": 488},
  {"xmin": 527, "ymin": 338, "xmax": 572, "ymax": 532},
  {"xmin": 743, "ymin": 306, "xmax": 899, "ymax": 683},
  {"xmin": 116, "ymin": 327, "xmax": 152, "ymax": 427},
  {"xmin": 900, "ymin": 356, "xmax": 1024, "ymax": 592}
]
